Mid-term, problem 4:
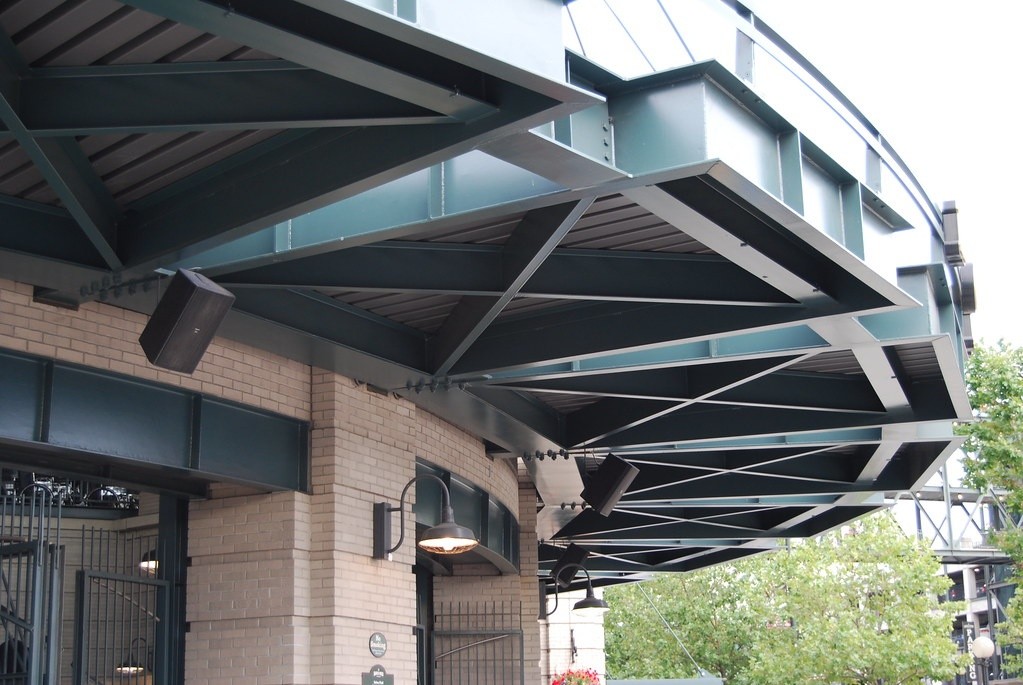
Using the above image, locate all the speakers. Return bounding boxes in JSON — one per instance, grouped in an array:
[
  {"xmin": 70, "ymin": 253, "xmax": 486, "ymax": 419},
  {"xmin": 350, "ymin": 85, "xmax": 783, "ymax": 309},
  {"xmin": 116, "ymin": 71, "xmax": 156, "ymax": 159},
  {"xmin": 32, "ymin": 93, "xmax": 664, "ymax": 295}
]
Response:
[
  {"xmin": 139, "ymin": 268, "xmax": 236, "ymax": 375},
  {"xmin": 550, "ymin": 543, "xmax": 590, "ymax": 588},
  {"xmin": 579, "ymin": 451, "xmax": 640, "ymax": 519}
]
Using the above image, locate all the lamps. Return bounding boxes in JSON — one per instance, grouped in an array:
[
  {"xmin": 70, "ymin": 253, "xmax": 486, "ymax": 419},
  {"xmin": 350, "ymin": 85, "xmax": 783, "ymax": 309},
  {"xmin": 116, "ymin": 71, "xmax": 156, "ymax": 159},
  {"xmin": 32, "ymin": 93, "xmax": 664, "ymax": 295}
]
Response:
[
  {"xmin": 138, "ymin": 550, "xmax": 158, "ymax": 574},
  {"xmin": 549, "ymin": 543, "xmax": 590, "ymax": 588},
  {"xmin": 116, "ymin": 637, "xmax": 153, "ymax": 674},
  {"xmin": 578, "ymin": 453, "xmax": 640, "ymax": 518},
  {"xmin": 138, "ymin": 268, "xmax": 235, "ymax": 375},
  {"xmin": 538, "ymin": 564, "xmax": 609, "ymax": 620},
  {"xmin": 372, "ymin": 474, "xmax": 477, "ymax": 562}
]
